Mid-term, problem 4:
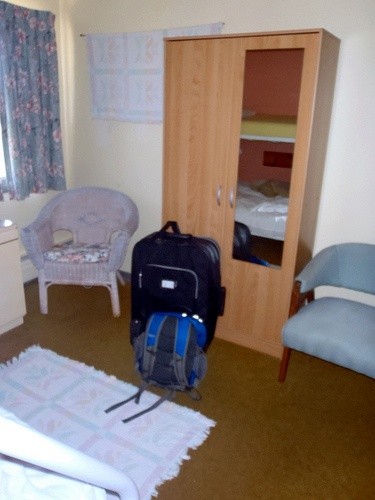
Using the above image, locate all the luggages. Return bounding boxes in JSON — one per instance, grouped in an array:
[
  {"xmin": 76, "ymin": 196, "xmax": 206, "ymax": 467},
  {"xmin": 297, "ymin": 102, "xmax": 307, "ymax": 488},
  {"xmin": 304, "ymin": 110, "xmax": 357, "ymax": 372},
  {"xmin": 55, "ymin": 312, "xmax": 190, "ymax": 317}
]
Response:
[{"xmin": 129, "ymin": 221, "xmax": 226, "ymax": 348}]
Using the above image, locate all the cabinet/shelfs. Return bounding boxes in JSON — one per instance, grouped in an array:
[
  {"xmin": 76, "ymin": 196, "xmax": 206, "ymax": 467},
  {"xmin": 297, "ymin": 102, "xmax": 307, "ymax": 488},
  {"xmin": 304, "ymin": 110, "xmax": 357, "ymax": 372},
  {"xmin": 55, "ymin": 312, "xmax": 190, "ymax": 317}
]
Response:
[{"xmin": 163, "ymin": 29, "xmax": 340, "ymax": 360}]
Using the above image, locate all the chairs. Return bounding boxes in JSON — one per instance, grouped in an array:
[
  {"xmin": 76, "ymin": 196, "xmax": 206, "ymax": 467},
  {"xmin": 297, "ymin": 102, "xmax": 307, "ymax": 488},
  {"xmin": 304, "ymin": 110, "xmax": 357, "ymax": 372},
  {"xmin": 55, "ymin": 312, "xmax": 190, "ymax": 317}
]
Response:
[
  {"xmin": 278, "ymin": 242, "xmax": 375, "ymax": 386},
  {"xmin": 21, "ymin": 186, "xmax": 139, "ymax": 318}
]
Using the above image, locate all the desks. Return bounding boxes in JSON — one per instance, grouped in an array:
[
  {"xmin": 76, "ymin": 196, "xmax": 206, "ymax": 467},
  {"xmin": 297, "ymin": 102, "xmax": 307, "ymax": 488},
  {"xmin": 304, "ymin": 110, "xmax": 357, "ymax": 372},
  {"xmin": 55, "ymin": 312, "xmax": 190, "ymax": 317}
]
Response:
[{"xmin": 1, "ymin": 227, "xmax": 25, "ymax": 335}]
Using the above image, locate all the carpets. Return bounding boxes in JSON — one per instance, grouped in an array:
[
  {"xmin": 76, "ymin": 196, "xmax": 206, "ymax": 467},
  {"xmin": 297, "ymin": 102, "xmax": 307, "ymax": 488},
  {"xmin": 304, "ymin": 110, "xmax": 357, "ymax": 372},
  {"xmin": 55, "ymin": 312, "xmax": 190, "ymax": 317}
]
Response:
[{"xmin": 0, "ymin": 345, "xmax": 218, "ymax": 500}]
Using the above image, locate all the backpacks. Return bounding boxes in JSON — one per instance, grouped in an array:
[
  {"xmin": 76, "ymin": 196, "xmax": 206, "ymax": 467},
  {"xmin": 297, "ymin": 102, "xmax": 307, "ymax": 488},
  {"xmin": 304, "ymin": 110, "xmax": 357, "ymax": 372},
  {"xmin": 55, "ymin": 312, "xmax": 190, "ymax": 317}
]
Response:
[{"xmin": 134, "ymin": 312, "xmax": 207, "ymax": 391}]
